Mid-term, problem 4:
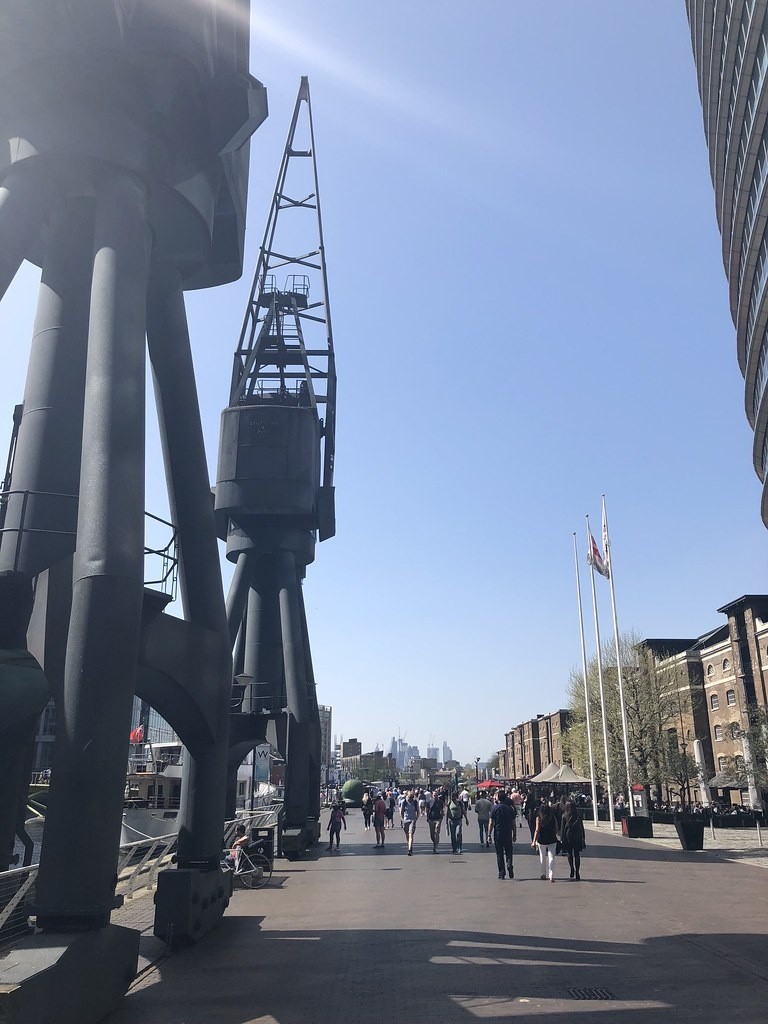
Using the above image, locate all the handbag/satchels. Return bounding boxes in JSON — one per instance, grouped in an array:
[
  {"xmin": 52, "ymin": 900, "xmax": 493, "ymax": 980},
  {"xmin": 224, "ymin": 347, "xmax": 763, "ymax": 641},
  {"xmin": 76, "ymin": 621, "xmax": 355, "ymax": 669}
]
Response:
[{"xmin": 581, "ymin": 838, "xmax": 585, "ymax": 849}]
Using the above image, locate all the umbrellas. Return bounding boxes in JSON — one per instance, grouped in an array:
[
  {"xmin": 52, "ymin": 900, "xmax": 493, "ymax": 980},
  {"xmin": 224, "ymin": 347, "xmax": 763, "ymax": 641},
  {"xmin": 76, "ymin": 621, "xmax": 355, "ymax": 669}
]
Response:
[
  {"xmin": 526, "ymin": 760, "xmax": 599, "ymax": 800},
  {"xmin": 477, "ymin": 779, "xmax": 505, "ymax": 795}
]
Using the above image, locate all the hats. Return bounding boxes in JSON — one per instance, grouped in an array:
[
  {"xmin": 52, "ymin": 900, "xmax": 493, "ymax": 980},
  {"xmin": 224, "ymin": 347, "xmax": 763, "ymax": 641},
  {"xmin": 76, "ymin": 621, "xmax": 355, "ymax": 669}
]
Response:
[{"xmin": 332, "ymin": 802, "xmax": 339, "ymax": 807}]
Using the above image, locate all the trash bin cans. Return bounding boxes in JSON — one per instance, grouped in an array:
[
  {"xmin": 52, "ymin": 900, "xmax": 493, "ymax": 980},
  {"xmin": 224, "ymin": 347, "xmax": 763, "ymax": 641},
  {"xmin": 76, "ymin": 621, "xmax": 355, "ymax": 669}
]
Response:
[
  {"xmin": 250, "ymin": 827, "xmax": 275, "ymax": 871},
  {"xmin": 338, "ymin": 800, "xmax": 346, "ymax": 816}
]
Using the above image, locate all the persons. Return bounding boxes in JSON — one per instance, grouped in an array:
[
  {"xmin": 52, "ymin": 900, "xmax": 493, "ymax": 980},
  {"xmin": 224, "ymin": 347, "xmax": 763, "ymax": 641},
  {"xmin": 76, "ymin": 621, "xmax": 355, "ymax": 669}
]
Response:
[
  {"xmin": 223, "ymin": 824, "xmax": 250, "ymax": 863},
  {"xmin": 321, "ymin": 780, "xmax": 755, "ymax": 883},
  {"xmin": 38, "ymin": 766, "xmax": 51, "ymax": 784}
]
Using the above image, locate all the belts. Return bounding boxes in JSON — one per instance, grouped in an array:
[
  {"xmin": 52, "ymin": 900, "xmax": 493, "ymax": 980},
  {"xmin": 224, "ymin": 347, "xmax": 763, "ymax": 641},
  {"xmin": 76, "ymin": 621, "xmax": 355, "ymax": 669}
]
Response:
[{"xmin": 450, "ymin": 817, "xmax": 463, "ymax": 820}]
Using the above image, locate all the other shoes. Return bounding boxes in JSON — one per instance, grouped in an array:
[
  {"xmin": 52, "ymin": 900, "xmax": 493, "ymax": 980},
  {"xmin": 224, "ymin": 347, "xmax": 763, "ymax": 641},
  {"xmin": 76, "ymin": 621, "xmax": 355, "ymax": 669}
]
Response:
[
  {"xmin": 480, "ymin": 843, "xmax": 484, "ymax": 847},
  {"xmin": 541, "ymin": 875, "xmax": 546, "ymax": 879},
  {"xmin": 576, "ymin": 875, "xmax": 580, "ymax": 879},
  {"xmin": 337, "ymin": 848, "xmax": 340, "ymax": 852},
  {"xmin": 407, "ymin": 849, "xmax": 412, "ymax": 856},
  {"xmin": 368, "ymin": 827, "xmax": 370, "ymax": 830},
  {"xmin": 365, "ymin": 828, "xmax": 368, "ymax": 831},
  {"xmin": 433, "ymin": 847, "xmax": 439, "ymax": 854},
  {"xmin": 372, "ymin": 845, "xmax": 384, "ymax": 849},
  {"xmin": 392, "ymin": 824, "xmax": 394, "ymax": 828},
  {"xmin": 454, "ymin": 848, "xmax": 462, "ymax": 855},
  {"xmin": 499, "ymin": 875, "xmax": 504, "ymax": 879},
  {"xmin": 486, "ymin": 844, "xmax": 489, "ymax": 847},
  {"xmin": 508, "ymin": 866, "xmax": 513, "ymax": 878},
  {"xmin": 326, "ymin": 847, "xmax": 333, "ymax": 851},
  {"xmin": 570, "ymin": 867, "xmax": 574, "ymax": 878},
  {"xmin": 550, "ymin": 878, "xmax": 554, "ymax": 883}
]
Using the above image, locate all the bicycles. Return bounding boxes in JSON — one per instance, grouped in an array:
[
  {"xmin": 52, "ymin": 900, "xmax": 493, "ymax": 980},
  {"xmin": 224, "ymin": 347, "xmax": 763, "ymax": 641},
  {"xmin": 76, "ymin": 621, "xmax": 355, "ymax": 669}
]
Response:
[{"xmin": 208, "ymin": 840, "xmax": 273, "ymax": 888}]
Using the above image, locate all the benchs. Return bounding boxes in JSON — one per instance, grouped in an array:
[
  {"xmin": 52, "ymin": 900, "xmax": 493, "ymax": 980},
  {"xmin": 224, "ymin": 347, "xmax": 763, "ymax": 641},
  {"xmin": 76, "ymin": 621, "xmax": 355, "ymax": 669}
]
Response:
[{"xmin": 223, "ymin": 838, "xmax": 264, "ymax": 887}]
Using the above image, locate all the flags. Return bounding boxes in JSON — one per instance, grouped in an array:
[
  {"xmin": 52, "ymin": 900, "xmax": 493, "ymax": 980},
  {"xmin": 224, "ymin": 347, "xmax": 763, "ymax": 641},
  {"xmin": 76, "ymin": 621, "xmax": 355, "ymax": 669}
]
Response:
[
  {"xmin": 585, "ymin": 496, "xmax": 612, "ymax": 579},
  {"xmin": 129, "ymin": 722, "xmax": 145, "ymax": 744}
]
